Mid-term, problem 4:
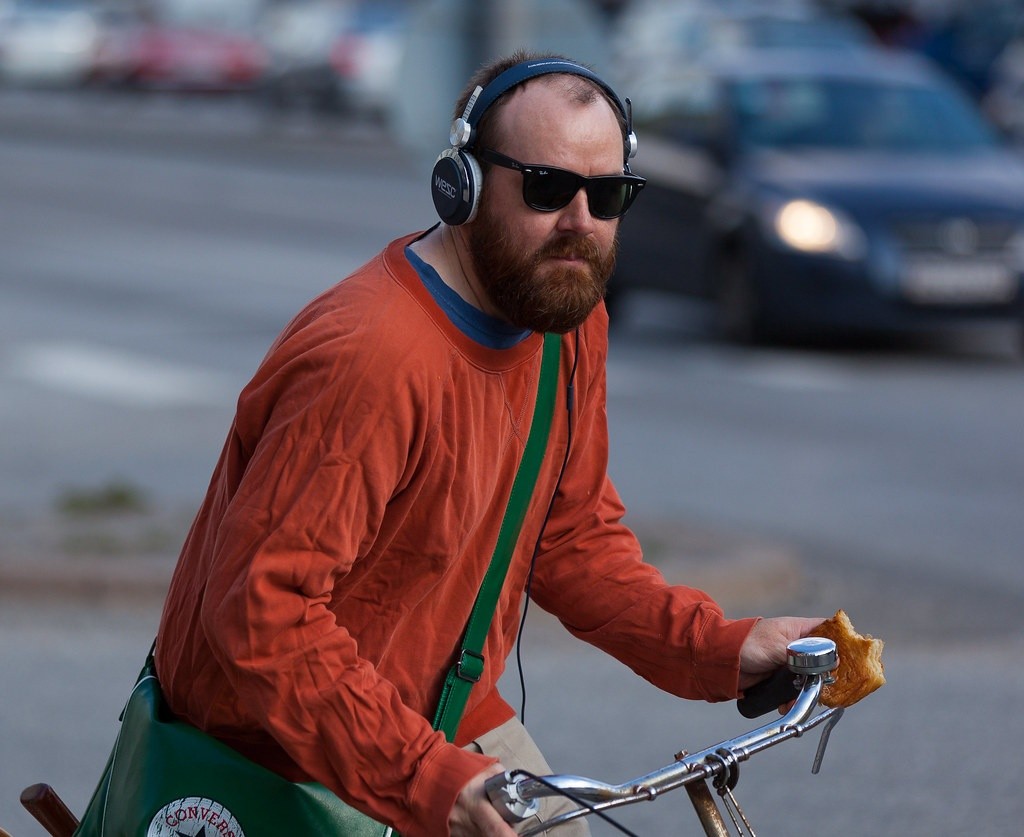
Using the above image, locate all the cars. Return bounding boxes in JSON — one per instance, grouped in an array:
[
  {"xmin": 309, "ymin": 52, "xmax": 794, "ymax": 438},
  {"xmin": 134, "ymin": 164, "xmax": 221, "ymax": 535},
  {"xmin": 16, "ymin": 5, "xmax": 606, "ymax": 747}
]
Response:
[{"xmin": 605, "ymin": 16, "xmax": 1022, "ymax": 352}]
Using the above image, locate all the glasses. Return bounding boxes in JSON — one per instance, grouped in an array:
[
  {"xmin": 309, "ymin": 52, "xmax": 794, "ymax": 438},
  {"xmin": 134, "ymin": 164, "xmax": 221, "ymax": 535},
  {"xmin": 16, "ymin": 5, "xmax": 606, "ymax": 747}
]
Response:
[{"xmin": 467, "ymin": 144, "xmax": 649, "ymax": 220}]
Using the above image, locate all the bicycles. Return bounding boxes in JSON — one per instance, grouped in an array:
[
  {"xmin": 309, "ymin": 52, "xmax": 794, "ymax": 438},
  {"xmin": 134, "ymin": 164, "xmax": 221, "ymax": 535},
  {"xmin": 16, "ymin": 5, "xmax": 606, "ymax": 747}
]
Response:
[{"xmin": 484, "ymin": 635, "xmax": 847, "ymax": 837}]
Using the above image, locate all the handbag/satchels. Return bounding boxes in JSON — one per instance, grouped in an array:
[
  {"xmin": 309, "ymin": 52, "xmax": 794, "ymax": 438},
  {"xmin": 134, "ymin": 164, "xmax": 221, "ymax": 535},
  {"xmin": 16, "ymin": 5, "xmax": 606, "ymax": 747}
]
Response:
[{"xmin": 65, "ymin": 654, "xmax": 404, "ymax": 837}]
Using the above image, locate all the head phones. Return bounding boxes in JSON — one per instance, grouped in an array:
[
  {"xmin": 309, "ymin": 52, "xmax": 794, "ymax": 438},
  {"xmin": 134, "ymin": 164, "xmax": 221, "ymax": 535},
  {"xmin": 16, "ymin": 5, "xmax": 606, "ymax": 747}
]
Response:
[{"xmin": 431, "ymin": 59, "xmax": 636, "ymax": 227}]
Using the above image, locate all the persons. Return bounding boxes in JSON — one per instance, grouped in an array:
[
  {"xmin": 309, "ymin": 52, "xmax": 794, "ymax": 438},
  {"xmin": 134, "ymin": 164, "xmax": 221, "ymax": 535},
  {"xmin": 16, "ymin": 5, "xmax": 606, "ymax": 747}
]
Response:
[{"xmin": 78, "ymin": 50, "xmax": 834, "ymax": 836}]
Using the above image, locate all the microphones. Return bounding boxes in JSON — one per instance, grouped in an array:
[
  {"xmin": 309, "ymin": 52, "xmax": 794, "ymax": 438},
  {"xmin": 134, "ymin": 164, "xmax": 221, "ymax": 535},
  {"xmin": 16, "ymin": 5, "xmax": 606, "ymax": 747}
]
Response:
[{"xmin": 624, "ymin": 96, "xmax": 637, "ymax": 159}]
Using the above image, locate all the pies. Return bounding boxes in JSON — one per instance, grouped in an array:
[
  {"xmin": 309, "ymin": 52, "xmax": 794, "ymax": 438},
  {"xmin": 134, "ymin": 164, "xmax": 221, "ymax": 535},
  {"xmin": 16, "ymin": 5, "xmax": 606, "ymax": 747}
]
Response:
[{"xmin": 804, "ymin": 609, "xmax": 886, "ymax": 708}]
